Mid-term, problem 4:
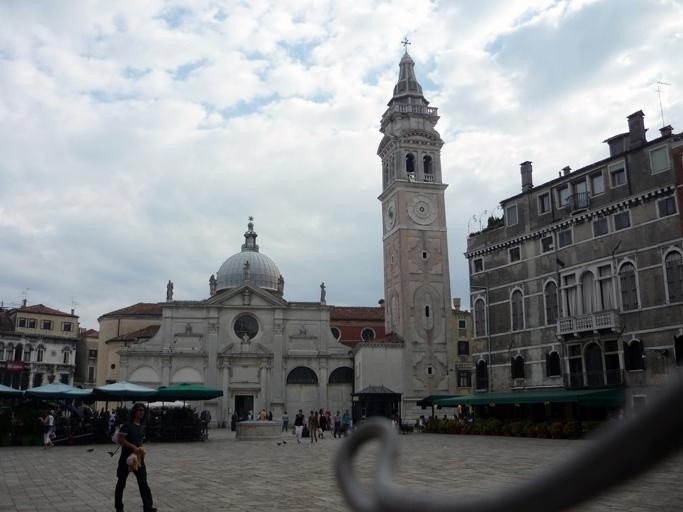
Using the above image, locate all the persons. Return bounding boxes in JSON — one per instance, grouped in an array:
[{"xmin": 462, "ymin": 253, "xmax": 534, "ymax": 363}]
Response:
[
  {"xmin": 231, "ymin": 407, "xmax": 272, "ymax": 431},
  {"xmin": 114, "ymin": 403, "xmax": 158, "ymax": 512},
  {"xmin": 38, "ymin": 408, "xmax": 55, "ymax": 450},
  {"xmin": 390, "ymin": 407, "xmax": 449, "ymax": 434},
  {"xmin": 282, "ymin": 405, "xmax": 367, "ymax": 449}
]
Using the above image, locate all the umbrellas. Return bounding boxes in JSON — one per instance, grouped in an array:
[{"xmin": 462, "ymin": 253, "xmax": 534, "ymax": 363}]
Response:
[
  {"xmin": 23, "ymin": 381, "xmax": 93, "ymax": 410},
  {"xmin": 0, "ymin": 384, "xmax": 23, "ymax": 398},
  {"xmin": 156, "ymin": 384, "xmax": 224, "ymax": 407},
  {"xmin": 93, "ymin": 381, "xmax": 157, "ymax": 408}
]
